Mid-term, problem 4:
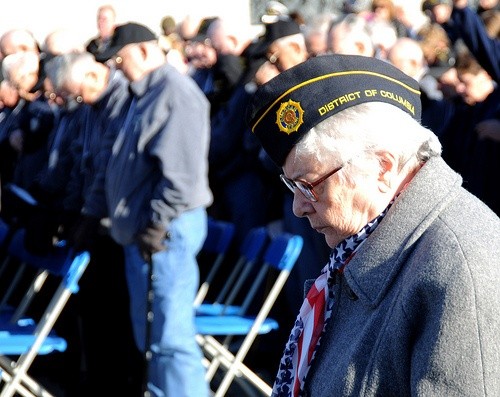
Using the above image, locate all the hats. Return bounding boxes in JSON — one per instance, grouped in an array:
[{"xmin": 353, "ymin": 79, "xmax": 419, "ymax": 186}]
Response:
[
  {"xmin": 98, "ymin": 22, "xmax": 155, "ymax": 61},
  {"xmin": 249, "ymin": 55, "xmax": 423, "ymax": 168},
  {"xmin": 258, "ymin": 19, "xmax": 301, "ymax": 52}
]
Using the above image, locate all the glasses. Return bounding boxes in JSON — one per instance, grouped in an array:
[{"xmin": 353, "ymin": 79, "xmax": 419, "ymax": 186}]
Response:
[{"xmin": 280, "ymin": 143, "xmax": 378, "ymax": 203}]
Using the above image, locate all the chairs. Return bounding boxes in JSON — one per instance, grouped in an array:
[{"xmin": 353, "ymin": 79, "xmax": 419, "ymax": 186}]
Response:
[
  {"xmin": 0, "ymin": 219, "xmax": 91, "ymax": 397},
  {"xmin": 193, "ymin": 218, "xmax": 303, "ymax": 397}
]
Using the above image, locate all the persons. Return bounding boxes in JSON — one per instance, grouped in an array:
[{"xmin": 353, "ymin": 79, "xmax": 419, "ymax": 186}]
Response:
[
  {"xmin": 251, "ymin": 52, "xmax": 500, "ymax": 397},
  {"xmin": 0, "ymin": 1, "xmax": 500, "ymax": 272},
  {"xmin": 95, "ymin": 22, "xmax": 214, "ymax": 396}
]
